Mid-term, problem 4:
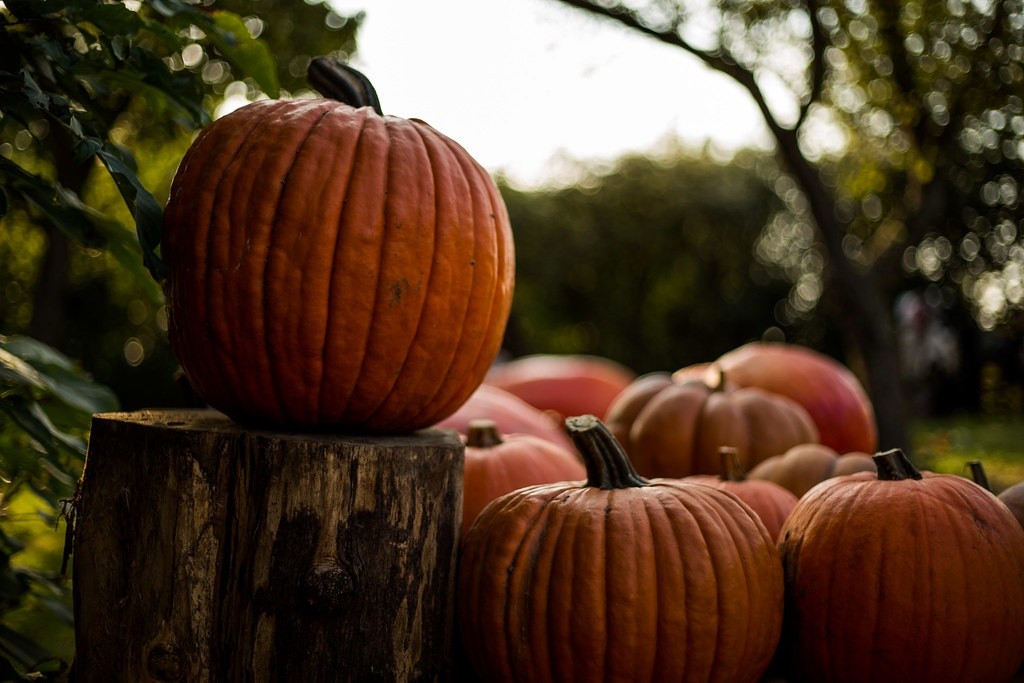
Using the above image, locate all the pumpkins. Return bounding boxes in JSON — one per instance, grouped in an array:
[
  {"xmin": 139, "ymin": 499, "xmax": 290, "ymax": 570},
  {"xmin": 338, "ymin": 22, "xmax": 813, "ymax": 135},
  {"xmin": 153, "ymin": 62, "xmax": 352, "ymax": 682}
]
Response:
[
  {"xmin": 164, "ymin": 57, "xmax": 514, "ymax": 438},
  {"xmin": 430, "ymin": 342, "xmax": 1024, "ymax": 683}
]
